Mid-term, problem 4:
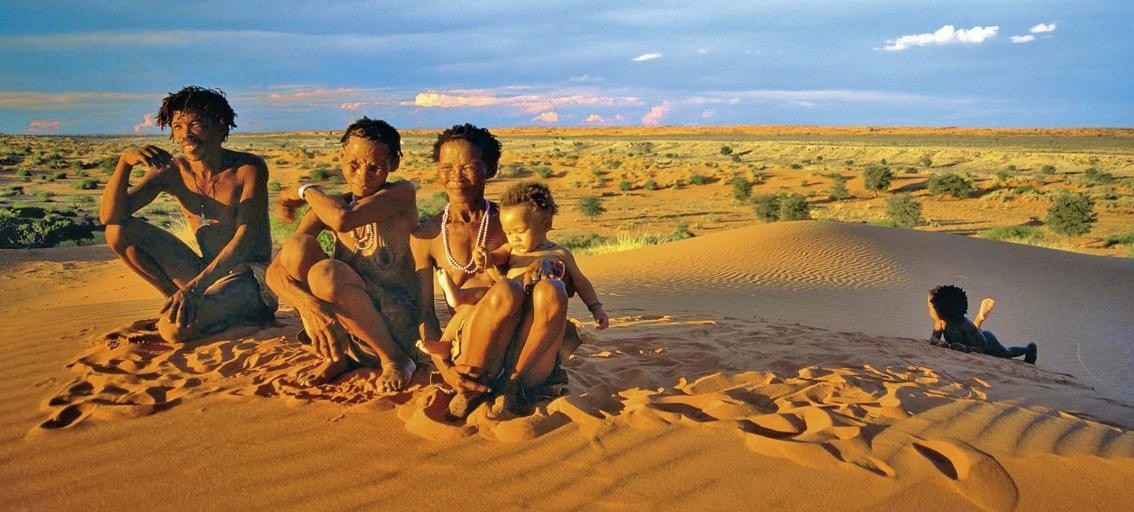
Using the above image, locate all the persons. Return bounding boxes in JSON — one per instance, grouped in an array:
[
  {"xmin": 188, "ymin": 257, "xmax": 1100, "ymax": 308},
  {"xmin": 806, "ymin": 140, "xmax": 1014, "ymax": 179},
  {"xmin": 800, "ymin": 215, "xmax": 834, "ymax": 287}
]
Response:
[
  {"xmin": 926, "ymin": 284, "xmax": 1036, "ymax": 365},
  {"xmin": 99, "ymin": 86, "xmax": 279, "ymax": 342},
  {"xmin": 265, "ymin": 115, "xmax": 421, "ymax": 392},
  {"xmin": 408, "ymin": 123, "xmax": 583, "ymax": 424},
  {"xmin": 415, "ymin": 180, "xmax": 612, "ymax": 360}
]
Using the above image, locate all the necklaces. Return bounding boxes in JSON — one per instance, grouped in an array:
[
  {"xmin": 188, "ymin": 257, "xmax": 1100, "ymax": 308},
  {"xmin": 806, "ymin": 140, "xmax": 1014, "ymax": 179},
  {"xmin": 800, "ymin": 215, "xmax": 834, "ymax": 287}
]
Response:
[
  {"xmin": 536, "ymin": 240, "xmax": 556, "ymax": 249},
  {"xmin": 442, "ymin": 197, "xmax": 490, "ymax": 270},
  {"xmin": 192, "ymin": 147, "xmax": 227, "ymax": 227},
  {"xmin": 439, "ymin": 198, "xmax": 489, "ymax": 276},
  {"xmin": 347, "ymin": 190, "xmax": 375, "ymax": 258}
]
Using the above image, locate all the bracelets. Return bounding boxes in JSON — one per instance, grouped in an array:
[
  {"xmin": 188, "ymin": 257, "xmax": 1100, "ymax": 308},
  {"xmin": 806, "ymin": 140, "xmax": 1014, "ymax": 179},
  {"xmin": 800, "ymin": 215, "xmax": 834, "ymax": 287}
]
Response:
[
  {"xmin": 295, "ymin": 181, "xmax": 325, "ymax": 201},
  {"xmin": 587, "ymin": 301, "xmax": 603, "ymax": 311},
  {"xmin": 546, "ymin": 259, "xmax": 566, "ymax": 281}
]
